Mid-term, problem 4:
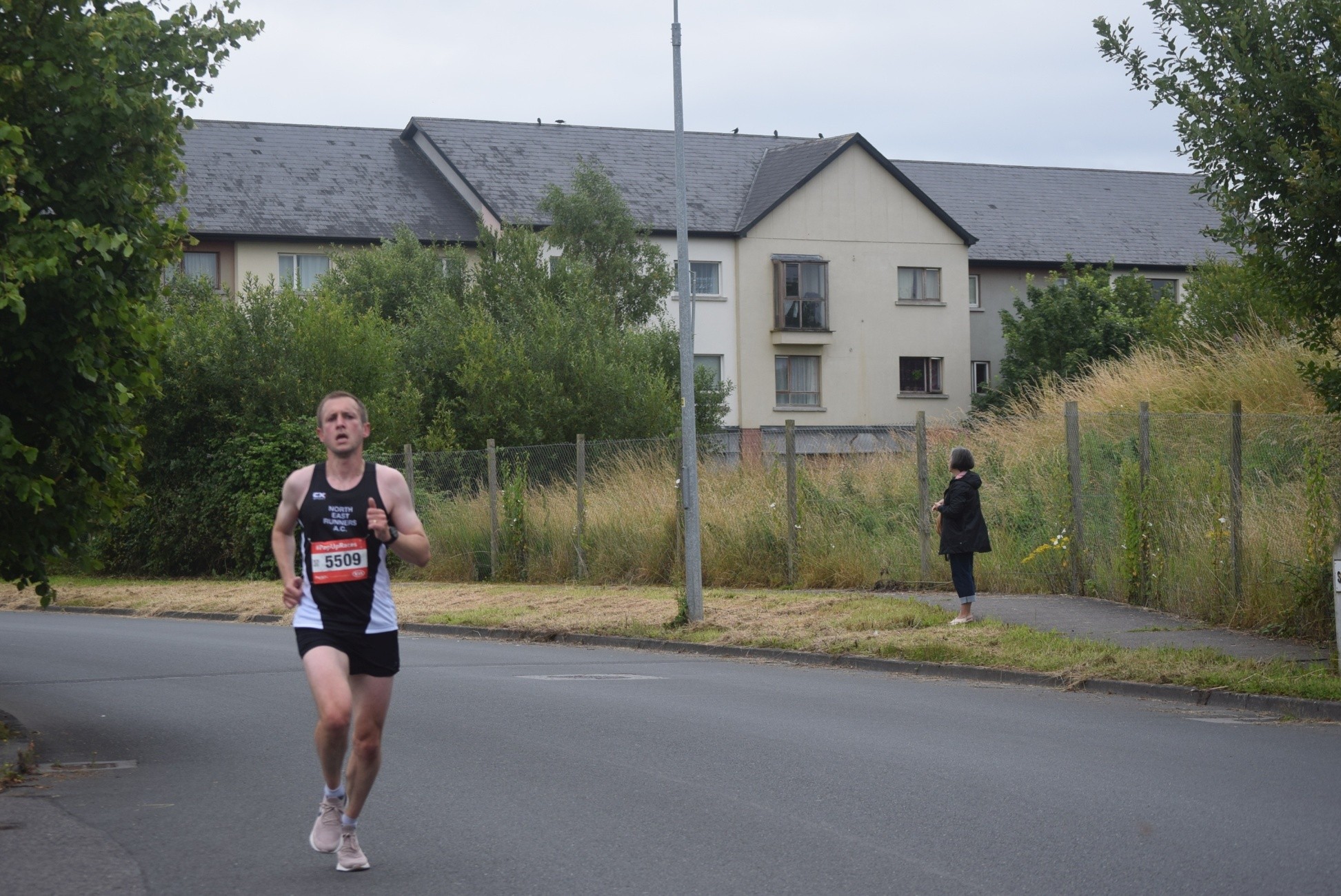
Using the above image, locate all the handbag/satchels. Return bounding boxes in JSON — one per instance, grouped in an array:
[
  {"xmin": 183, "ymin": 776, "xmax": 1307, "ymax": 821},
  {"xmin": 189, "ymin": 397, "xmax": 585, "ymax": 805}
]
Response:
[{"xmin": 936, "ymin": 513, "xmax": 942, "ymax": 535}]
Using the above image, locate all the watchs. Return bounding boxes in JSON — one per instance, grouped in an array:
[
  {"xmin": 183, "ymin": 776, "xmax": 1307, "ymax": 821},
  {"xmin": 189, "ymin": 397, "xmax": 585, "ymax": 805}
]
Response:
[{"xmin": 382, "ymin": 526, "xmax": 399, "ymax": 544}]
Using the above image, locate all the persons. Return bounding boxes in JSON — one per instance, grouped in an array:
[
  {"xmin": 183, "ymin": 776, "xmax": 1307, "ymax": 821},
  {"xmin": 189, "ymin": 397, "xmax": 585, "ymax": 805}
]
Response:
[
  {"xmin": 932, "ymin": 448, "xmax": 992, "ymax": 625},
  {"xmin": 272, "ymin": 391, "xmax": 432, "ymax": 873}
]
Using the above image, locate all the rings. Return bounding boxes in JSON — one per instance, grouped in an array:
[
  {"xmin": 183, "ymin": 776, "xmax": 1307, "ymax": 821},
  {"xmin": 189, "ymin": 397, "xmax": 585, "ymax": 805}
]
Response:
[{"xmin": 374, "ymin": 519, "xmax": 376, "ymax": 523}]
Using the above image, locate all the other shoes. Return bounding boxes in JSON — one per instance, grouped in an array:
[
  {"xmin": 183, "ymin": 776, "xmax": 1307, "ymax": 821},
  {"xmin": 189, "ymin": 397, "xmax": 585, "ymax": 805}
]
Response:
[{"xmin": 948, "ymin": 615, "xmax": 973, "ymax": 626}]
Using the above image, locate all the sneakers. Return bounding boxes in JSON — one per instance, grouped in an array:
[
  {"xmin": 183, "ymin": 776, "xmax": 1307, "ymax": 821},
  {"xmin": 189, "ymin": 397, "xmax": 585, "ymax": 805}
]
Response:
[
  {"xmin": 336, "ymin": 824, "xmax": 370, "ymax": 872},
  {"xmin": 311, "ymin": 795, "xmax": 347, "ymax": 853}
]
